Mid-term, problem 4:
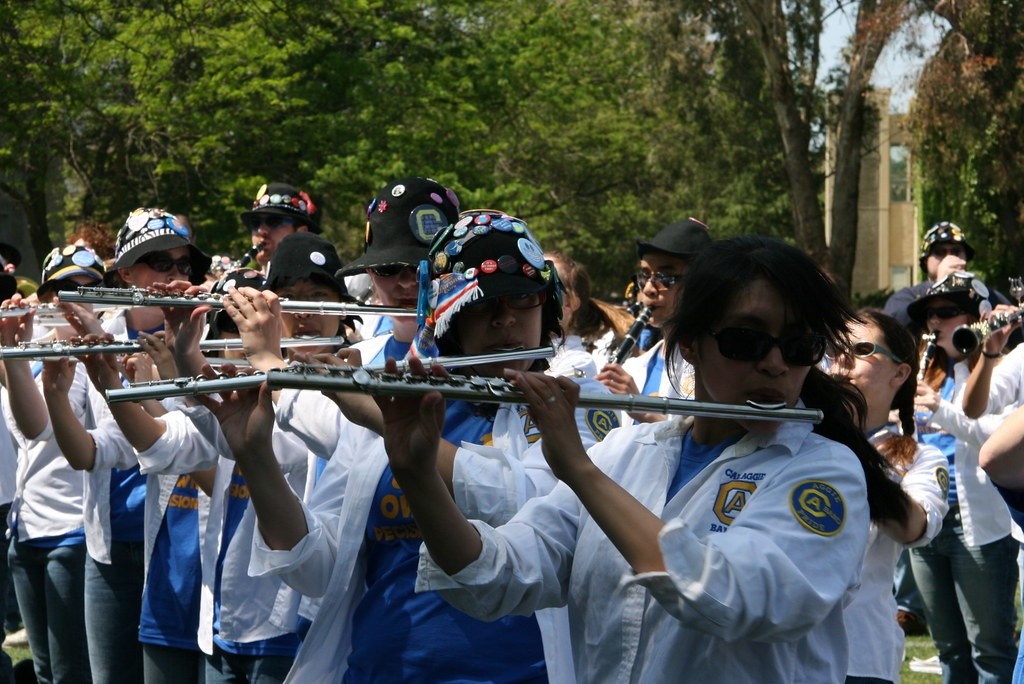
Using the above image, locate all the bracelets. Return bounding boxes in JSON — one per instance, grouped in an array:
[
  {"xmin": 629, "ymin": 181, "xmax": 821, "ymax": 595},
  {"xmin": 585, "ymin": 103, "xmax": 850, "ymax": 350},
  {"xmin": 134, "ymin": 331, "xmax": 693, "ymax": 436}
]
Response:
[{"xmin": 980, "ymin": 350, "xmax": 1000, "ymax": 358}]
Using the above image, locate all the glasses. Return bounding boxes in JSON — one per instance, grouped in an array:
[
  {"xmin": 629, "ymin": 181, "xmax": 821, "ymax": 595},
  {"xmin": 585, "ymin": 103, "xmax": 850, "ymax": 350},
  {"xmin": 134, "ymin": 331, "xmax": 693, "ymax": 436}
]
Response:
[
  {"xmin": 925, "ymin": 306, "xmax": 966, "ymax": 319},
  {"xmin": 825, "ymin": 343, "xmax": 904, "ymax": 364},
  {"xmin": 372, "ymin": 266, "xmax": 417, "ymax": 276},
  {"xmin": 245, "ymin": 216, "xmax": 298, "ymax": 231},
  {"xmin": 701, "ymin": 324, "xmax": 827, "ymax": 367},
  {"xmin": 932, "ymin": 247, "xmax": 963, "ymax": 256},
  {"xmin": 637, "ymin": 273, "xmax": 684, "ymax": 288},
  {"xmin": 462, "ymin": 288, "xmax": 547, "ymax": 315},
  {"xmin": 53, "ymin": 279, "xmax": 103, "ymax": 297},
  {"xmin": 144, "ymin": 252, "xmax": 194, "ymax": 276}
]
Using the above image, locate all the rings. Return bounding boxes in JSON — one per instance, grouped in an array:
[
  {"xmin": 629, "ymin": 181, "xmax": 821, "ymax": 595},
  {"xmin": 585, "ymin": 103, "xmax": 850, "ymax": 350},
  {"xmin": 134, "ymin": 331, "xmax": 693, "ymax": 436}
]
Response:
[{"xmin": 546, "ymin": 395, "xmax": 557, "ymax": 404}]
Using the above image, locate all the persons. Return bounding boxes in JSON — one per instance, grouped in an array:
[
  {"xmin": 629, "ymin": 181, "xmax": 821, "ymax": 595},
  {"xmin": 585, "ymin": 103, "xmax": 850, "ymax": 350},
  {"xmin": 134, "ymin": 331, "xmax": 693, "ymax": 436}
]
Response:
[
  {"xmin": 1, "ymin": 178, "xmax": 1024, "ymax": 684},
  {"xmin": 193, "ymin": 208, "xmax": 623, "ymax": 684},
  {"xmin": 827, "ymin": 310, "xmax": 951, "ymax": 684},
  {"xmin": 370, "ymin": 233, "xmax": 912, "ymax": 684}
]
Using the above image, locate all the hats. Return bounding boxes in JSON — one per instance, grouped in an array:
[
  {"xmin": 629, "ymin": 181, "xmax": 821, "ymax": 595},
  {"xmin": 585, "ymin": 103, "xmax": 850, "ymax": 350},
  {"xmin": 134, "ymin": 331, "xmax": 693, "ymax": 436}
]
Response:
[
  {"xmin": 38, "ymin": 241, "xmax": 107, "ymax": 303},
  {"xmin": 906, "ymin": 273, "xmax": 986, "ymax": 326},
  {"xmin": 109, "ymin": 207, "xmax": 212, "ymax": 280},
  {"xmin": 209, "ymin": 269, "xmax": 267, "ymax": 333},
  {"xmin": 241, "ymin": 184, "xmax": 323, "ymax": 234},
  {"xmin": 919, "ymin": 222, "xmax": 976, "ymax": 273},
  {"xmin": 254, "ymin": 232, "xmax": 355, "ymax": 301},
  {"xmin": 333, "ymin": 177, "xmax": 460, "ymax": 277},
  {"xmin": 432, "ymin": 209, "xmax": 554, "ymax": 318},
  {"xmin": 637, "ymin": 220, "xmax": 713, "ymax": 261}
]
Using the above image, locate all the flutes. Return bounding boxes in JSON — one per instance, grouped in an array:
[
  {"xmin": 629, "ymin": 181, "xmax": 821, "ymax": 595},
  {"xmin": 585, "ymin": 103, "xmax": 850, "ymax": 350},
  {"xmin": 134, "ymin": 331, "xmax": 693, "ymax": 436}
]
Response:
[
  {"xmin": 265, "ymin": 364, "xmax": 826, "ymax": 426},
  {"xmin": 358, "ymin": 283, "xmax": 375, "ymax": 302},
  {"xmin": 3, "ymin": 303, "xmax": 126, "ymax": 319},
  {"xmin": 57, "ymin": 285, "xmax": 421, "ymax": 319},
  {"xmin": 0, "ymin": 334, "xmax": 346, "ymax": 361},
  {"xmin": 918, "ymin": 329, "xmax": 941, "ymax": 392},
  {"xmin": 608, "ymin": 301, "xmax": 655, "ymax": 368},
  {"xmin": 232, "ymin": 240, "xmax": 267, "ymax": 267},
  {"xmin": 953, "ymin": 308, "xmax": 1018, "ymax": 356},
  {"xmin": 103, "ymin": 345, "xmax": 557, "ymax": 407}
]
